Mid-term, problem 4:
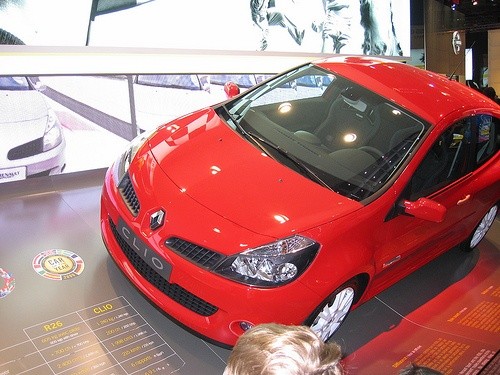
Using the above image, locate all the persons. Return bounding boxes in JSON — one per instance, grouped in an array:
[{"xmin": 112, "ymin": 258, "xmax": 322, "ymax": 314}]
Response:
[{"xmin": 222, "ymin": 322, "xmax": 350, "ymax": 375}]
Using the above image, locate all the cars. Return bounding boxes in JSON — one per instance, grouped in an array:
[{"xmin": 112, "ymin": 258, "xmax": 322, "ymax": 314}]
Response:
[
  {"xmin": 0, "ymin": 75, "xmax": 70, "ymax": 184},
  {"xmin": 101, "ymin": 57, "xmax": 500, "ymax": 350}
]
